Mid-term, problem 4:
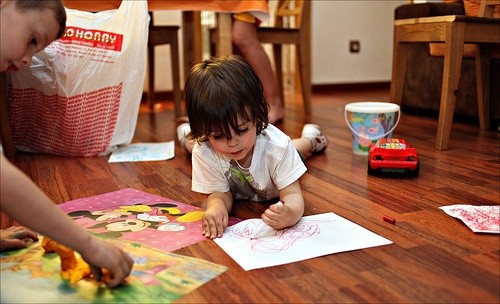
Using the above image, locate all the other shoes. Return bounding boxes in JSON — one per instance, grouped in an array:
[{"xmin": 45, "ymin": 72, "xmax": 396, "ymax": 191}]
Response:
[
  {"xmin": 176, "ymin": 122, "xmax": 195, "ymax": 146},
  {"xmin": 301, "ymin": 123, "xmax": 330, "ymax": 154}
]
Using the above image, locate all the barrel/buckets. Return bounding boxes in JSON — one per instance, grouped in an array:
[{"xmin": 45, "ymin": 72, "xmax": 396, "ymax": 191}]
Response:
[{"xmin": 343, "ymin": 101, "xmax": 401, "ymax": 156}]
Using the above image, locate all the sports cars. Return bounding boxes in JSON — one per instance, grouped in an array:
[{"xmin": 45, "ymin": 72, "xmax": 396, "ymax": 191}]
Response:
[{"xmin": 368, "ymin": 138, "xmax": 421, "ymax": 176}]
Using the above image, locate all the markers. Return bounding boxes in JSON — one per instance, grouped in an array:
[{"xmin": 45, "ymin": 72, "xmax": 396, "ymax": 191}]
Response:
[{"xmin": 250, "ymin": 200, "xmax": 285, "ymax": 240}]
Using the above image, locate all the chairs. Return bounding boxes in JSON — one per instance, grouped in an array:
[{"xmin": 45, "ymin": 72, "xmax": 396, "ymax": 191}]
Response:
[{"xmin": 206, "ymin": 0, "xmax": 314, "ymax": 124}]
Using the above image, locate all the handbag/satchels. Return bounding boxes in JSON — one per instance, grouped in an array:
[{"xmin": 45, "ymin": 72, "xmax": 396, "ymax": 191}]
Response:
[{"xmin": 5, "ymin": 1, "xmax": 150, "ymax": 157}]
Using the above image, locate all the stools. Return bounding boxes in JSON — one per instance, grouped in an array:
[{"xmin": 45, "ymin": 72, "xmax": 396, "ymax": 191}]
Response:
[{"xmin": 386, "ymin": 13, "xmax": 500, "ymax": 152}]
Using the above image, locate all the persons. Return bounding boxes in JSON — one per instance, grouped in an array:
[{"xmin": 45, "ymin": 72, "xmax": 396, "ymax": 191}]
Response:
[
  {"xmin": 231, "ymin": 0, "xmax": 289, "ymax": 126},
  {"xmin": 0, "ymin": 0, "xmax": 136, "ymax": 288},
  {"xmin": 176, "ymin": 55, "xmax": 328, "ymax": 243}
]
